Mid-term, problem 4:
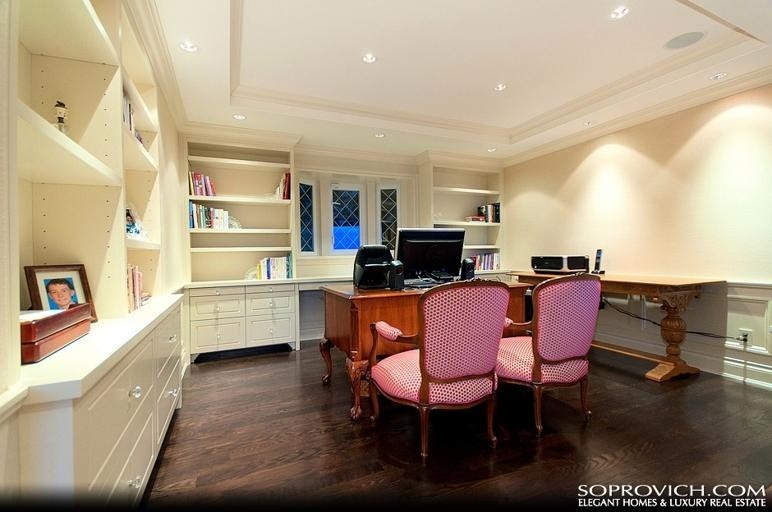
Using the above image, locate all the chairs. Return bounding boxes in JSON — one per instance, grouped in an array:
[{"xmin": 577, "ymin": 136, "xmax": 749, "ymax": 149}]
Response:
[{"xmin": 364, "ymin": 271, "xmax": 602, "ymax": 467}]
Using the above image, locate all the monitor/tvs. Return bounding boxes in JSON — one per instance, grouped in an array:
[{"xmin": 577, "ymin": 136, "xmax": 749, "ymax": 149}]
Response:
[{"xmin": 395, "ymin": 228, "xmax": 465, "ymax": 288}]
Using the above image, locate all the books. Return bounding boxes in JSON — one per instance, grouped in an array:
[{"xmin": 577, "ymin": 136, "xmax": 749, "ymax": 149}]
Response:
[
  {"xmin": 276, "ymin": 172, "xmax": 290, "ymax": 199},
  {"xmin": 255, "ymin": 255, "xmax": 292, "ymax": 280},
  {"xmin": 127, "ymin": 264, "xmax": 151, "ymax": 313},
  {"xmin": 467, "ymin": 251, "xmax": 498, "ymax": 271},
  {"xmin": 463, "ymin": 202, "xmax": 500, "ymax": 223},
  {"xmin": 188, "ymin": 171, "xmax": 216, "ymax": 196},
  {"xmin": 189, "ymin": 201, "xmax": 229, "ymax": 229}
]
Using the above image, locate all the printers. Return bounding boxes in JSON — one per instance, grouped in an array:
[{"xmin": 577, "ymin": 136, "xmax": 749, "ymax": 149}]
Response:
[{"xmin": 531, "ymin": 255, "xmax": 589, "ymax": 273}]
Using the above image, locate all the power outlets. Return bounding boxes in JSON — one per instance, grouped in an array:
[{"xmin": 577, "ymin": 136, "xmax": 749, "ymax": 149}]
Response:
[{"xmin": 738, "ymin": 329, "xmax": 753, "ymax": 345}]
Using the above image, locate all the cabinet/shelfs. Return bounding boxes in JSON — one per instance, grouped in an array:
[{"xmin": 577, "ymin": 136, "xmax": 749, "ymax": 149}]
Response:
[
  {"xmin": 184, "ymin": 139, "xmax": 301, "ymax": 368},
  {"xmin": 0, "ymin": 0, "xmax": 183, "ymax": 509},
  {"xmin": 418, "ymin": 164, "xmax": 504, "ymax": 275}
]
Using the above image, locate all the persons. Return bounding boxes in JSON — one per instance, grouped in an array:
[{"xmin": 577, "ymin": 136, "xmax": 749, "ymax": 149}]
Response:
[{"xmin": 46, "ymin": 278, "xmax": 78, "ymax": 309}]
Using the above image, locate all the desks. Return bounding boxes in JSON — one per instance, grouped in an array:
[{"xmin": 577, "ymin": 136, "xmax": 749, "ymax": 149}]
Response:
[
  {"xmin": 504, "ymin": 271, "xmax": 726, "ymax": 384},
  {"xmin": 319, "ymin": 281, "xmax": 534, "ymax": 418}
]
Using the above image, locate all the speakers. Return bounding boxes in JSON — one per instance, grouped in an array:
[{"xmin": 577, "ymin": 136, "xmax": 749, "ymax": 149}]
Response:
[
  {"xmin": 462, "ymin": 258, "xmax": 474, "ymax": 280},
  {"xmin": 390, "ymin": 261, "xmax": 405, "ymax": 290}
]
